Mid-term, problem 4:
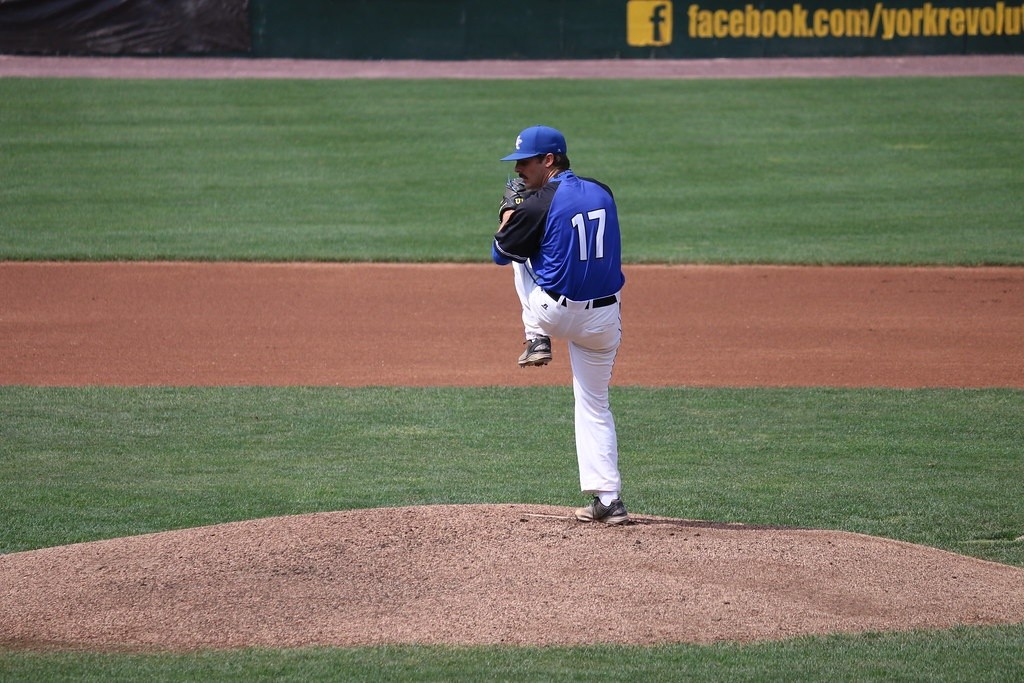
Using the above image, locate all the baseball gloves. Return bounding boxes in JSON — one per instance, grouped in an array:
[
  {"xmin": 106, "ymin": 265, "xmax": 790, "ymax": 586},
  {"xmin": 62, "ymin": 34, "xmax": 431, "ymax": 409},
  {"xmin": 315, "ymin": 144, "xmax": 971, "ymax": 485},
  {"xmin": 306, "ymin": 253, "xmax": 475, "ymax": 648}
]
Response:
[{"xmin": 497, "ymin": 170, "xmax": 539, "ymax": 224}]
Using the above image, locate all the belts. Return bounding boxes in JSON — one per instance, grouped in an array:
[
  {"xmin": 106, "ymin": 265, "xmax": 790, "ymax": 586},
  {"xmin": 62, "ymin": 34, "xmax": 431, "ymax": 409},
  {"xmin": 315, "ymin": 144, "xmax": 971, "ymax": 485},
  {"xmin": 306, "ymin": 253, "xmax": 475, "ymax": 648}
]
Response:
[{"xmin": 546, "ymin": 290, "xmax": 617, "ymax": 309}]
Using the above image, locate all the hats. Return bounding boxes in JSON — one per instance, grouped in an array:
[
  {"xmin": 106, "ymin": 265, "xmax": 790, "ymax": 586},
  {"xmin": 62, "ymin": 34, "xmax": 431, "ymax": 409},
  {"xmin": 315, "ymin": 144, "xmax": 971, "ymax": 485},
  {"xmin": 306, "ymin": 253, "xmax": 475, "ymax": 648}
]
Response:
[{"xmin": 499, "ymin": 125, "xmax": 567, "ymax": 161}]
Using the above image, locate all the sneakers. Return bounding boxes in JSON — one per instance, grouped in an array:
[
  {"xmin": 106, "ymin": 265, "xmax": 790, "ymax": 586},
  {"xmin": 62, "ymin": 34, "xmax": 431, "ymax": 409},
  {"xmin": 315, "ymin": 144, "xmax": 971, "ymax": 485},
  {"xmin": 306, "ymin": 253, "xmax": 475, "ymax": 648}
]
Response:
[
  {"xmin": 518, "ymin": 337, "xmax": 552, "ymax": 369},
  {"xmin": 575, "ymin": 496, "xmax": 628, "ymax": 524}
]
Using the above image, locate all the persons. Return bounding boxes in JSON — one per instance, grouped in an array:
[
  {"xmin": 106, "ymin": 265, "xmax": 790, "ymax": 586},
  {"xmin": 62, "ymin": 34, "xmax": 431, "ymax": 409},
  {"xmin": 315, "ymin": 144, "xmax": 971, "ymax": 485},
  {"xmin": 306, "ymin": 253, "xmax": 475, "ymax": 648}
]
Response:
[{"xmin": 492, "ymin": 124, "xmax": 629, "ymax": 526}]
